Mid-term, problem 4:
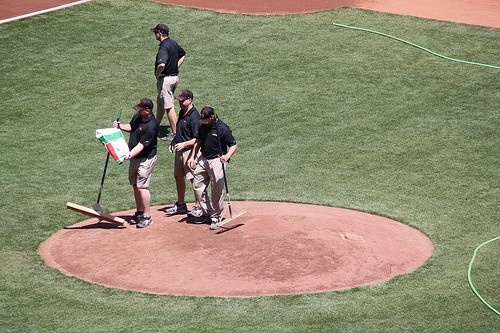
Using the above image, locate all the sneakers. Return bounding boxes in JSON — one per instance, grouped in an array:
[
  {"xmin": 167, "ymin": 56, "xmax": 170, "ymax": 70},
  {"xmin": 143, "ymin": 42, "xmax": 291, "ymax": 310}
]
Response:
[
  {"xmin": 127, "ymin": 211, "xmax": 152, "ymax": 228},
  {"xmin": 162, "ymin": 132, "xmax": 176, "ymax": 141},
  {"xmin": 187, "ymin": 205, "xmax": 203, "ymax": 217},
  {"xmin": 195, "ymin": 215, "xmax": 219, "ymax": 229},
  {"xmin": 166, "ymin": 202, "xmax": 188, "ymax": 215}
]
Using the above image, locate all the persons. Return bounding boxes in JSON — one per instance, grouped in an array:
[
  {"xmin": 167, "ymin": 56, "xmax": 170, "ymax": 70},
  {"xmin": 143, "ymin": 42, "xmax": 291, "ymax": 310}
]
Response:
[
  {"xmin": 165, "ymin": 89, "xmax": 203, "ymax": 217},
  {"xmin": 188, "ymin": 107, "xmax": 238, "ymax": 230},
  {"xmin": 112, "ymin": 98, "xmax": 158, "ymax": 229},
  {"xmin": 150, "ymin": 23, "xmax": 186, "ymax": 141}
]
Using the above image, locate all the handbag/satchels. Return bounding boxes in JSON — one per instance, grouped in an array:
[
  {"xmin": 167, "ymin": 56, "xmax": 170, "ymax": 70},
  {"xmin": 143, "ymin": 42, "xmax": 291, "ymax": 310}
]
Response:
[{"xmin": 96, "ymin": 128, "xmax": 130, "ymax": 164}]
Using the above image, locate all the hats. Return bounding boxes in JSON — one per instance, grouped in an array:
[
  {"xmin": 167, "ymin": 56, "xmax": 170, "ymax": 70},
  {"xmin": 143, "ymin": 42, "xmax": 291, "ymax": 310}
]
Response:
[
  {"xmin": 133, "ymin": 98, "xmax": 154, "ymax": 112},
  {"xmin": 174, "ymin": 90, "xmax": 195, "ymax": 102},
  {"xmin": 199, "ymin": 107, "xmax": 215, "ymax": 125},
  {"xmin": 150, "ymin": 24, "xmax": 169, "ymax": 35}
]
